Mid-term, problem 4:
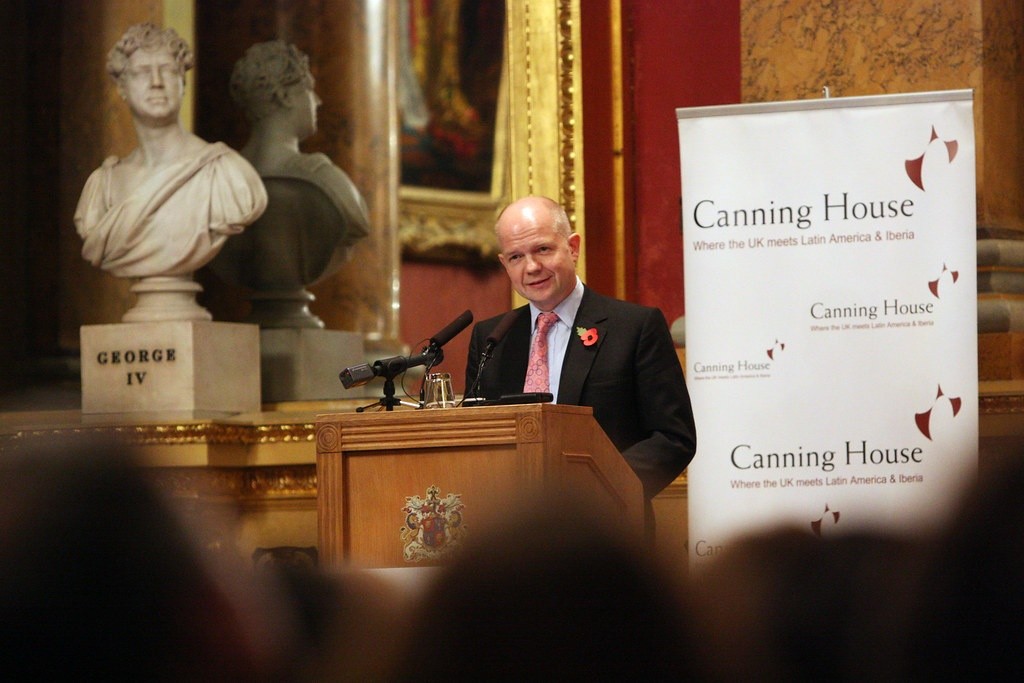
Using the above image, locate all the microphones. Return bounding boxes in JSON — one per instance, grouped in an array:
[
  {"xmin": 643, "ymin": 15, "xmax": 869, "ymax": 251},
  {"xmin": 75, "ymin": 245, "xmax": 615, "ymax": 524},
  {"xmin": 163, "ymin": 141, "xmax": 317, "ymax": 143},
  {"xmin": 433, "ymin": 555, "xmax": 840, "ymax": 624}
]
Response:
[
  {"xmin": 428, "ymin": 309, "xmax": 474, "ymax": 353},
  {"xmin": 486, "ymin": 307, "xmax": 522, "ymax": 346}
]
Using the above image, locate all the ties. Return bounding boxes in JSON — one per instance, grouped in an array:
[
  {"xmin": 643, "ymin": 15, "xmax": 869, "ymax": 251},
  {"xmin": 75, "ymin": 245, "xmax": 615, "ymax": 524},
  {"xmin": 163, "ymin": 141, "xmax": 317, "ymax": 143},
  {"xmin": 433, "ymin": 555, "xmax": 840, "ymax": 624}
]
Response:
[{"xmin": 523, "ymin": 313, "xmax": 561, "ymax": 405}]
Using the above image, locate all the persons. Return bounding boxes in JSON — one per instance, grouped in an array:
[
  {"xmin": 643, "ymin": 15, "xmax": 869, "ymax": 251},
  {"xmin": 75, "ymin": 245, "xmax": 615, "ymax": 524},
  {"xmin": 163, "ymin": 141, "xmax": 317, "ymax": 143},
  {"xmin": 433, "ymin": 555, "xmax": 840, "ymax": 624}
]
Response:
[
  {"xmin": 218, "ymin": 45, "xmax": 374, "ymax": 296},
  {"xmin": 78, "ymin": 22, "xmax": 272, "ymax": 281},
  {"xmin": 462, "ymin": 197, "xmax": 697, "ymax": 554}
]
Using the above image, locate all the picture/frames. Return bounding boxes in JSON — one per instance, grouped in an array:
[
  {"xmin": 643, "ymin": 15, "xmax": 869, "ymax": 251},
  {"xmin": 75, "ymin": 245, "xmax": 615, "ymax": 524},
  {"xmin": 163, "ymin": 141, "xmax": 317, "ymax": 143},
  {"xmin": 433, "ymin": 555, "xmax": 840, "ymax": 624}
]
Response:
[{"xmin": 195, "ymin": 0, "xmax": 513, "ymax": 266}]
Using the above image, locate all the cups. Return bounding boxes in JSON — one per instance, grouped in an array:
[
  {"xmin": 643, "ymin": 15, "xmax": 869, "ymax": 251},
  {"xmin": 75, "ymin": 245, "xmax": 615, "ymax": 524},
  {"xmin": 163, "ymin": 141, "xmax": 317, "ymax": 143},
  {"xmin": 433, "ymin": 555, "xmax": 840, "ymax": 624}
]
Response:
[{"xmin": 426, "ymin": 373, "xmax": 451, "ymax": 409}]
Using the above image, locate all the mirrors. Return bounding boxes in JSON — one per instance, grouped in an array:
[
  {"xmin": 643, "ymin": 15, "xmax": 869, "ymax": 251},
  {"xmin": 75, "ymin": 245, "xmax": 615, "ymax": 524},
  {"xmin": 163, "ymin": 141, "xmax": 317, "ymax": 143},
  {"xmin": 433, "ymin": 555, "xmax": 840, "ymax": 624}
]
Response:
[{"xmin": 192, "ymin": 0, "xmax": 531, "ymax": 400}]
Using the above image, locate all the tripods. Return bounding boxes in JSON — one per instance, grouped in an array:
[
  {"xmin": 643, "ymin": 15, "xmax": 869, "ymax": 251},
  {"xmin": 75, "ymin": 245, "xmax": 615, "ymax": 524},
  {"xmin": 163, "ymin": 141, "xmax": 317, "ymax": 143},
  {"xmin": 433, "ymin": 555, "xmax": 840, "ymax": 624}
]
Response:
[{"xmin": 355, "ymin": 377, "xmax": 424, "ymax": 414}]
